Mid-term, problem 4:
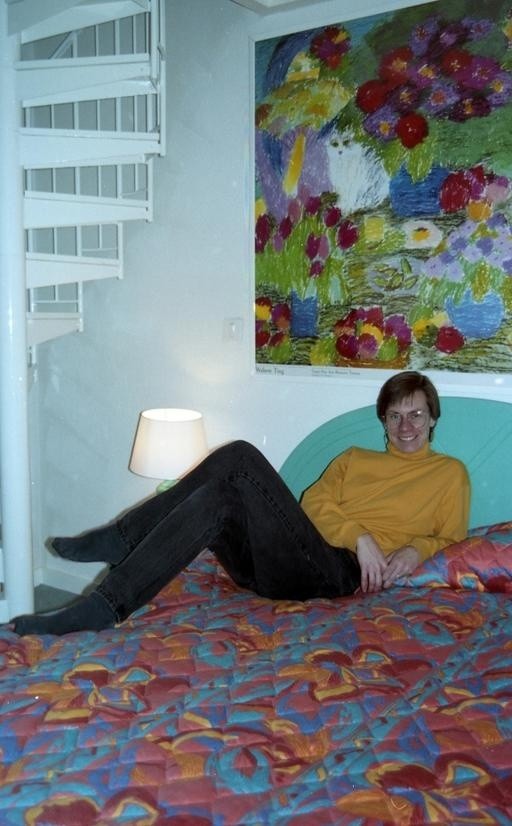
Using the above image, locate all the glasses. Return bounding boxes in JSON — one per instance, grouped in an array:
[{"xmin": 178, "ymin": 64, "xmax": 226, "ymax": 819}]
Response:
[{"xmin": 386, "ymin": 411, "xmax": 427, "ymax": 428}]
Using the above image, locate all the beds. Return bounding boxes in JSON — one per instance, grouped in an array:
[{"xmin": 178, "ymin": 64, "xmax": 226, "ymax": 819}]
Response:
[{"xmin": 2, "ymin": 397, "xmax": 510, "ymax": 825}]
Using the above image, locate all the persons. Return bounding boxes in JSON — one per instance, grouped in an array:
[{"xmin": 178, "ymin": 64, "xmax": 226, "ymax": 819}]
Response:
[{"xmin": 10, "ymin": 368, "xmax": 472, "ymax": 639}]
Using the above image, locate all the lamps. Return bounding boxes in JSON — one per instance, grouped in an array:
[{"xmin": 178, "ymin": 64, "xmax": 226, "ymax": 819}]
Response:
[{"xmin": 129, "ymin": 408, "xmax": 208, "ymax": 494}]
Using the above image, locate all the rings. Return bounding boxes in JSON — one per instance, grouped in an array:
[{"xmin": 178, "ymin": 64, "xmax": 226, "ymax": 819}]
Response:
[{"xmin": 397, "ymin": 574, "xmax": 403, "ymax": 578}]
